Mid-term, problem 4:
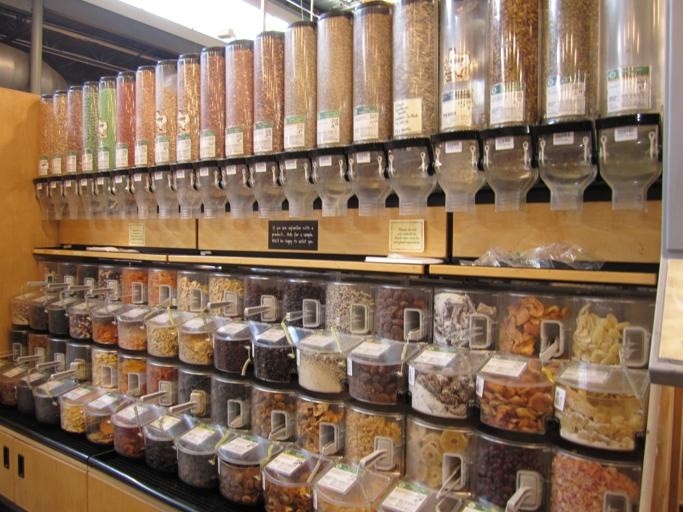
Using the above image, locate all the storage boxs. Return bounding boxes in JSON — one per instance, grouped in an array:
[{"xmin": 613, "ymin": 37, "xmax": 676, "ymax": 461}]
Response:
[
  {"xmin": 140, "ymin": 358, "xmax": 178, "ymax": 409},
  {"xmin": 282, "ymin": 277, "xmax": 324, "ymax": 328},
  {"xmin": 345, "ymin": 399, "xmax": 405, "ymax": 478},
  {"xmin": 407, "ymin": 347, "xmax": 478, "ymax": 425},
  {"xmin": 346, "ymin": 338, "xmax": 427, "ymax": 412},
  {"xmin": 59, "ymin": 290, "xmax": 104, "ymax": 343},
  {"xmin": 553, "ymin": 363, "xmax": 650, "ymax": 461},
  {"xmin": 33, "ymin": 378, "xmax": 81, "ymax": 423},
  {"xmin": 550, "ymin": 446, "xmax": 644, "ymax": 512},
  {"xmin": 16, "ymin": 372, "xmax": 50, "ymax": 412},
  {"xmin": 36, "ymin": 339, "xmax": 66, "ymax": 373},
  {"xmin": 475, "ymin": 355, "xmax": 553, "ymax": 440},
  {"xmin": 47, "ymin": 262, "xmax": 77, "ymax": 296},
  {"xmin": 10, "ymin": 292, "xmax": 45, "ymax": 330},
  {"xmin": 92, "ymin": 348, "xmax": 117, "ymax": 389},
  {"xmin": 116, "ymin": 353, "xmax": 146, "ymax": 397},
  {"xmin": 116, "ymin": 305, "xmax": 164, "ymax": 356},
  {"xmin": 148, "ymin": 266, "xmax": 176, "ymax": 309},
  {"xmin": 144, "ymin": 412, "xmax": 199, "ymax": 473},
  {"xmin": 84, "ymin": 392, "xmax": 136, "ymax": 445},
  {"xmin": 252, "ymin": 328, "xmax": 299, "ymax": 389},
  {"xmin": 90, "ymin": 265, "xmax": 120, "ymax": 301},
  {"xmin": 144, "ymin": 309, "xmax": 198, "ymax": 363},
  {"xmin": 314, "ymin": 463, "xmax": 400, "ymax": 512},
  {"xmin": 48, "ymin": 299, "xmax": 79, "ymax": 338},
  {"xmin": 175, "ymin": 421, "xmax": 239, "ymax": 488},
  {"xmin": 58, "ymin": 382, "xmax": 108, "ymax": 433},
  {"xmin": 242, "ymin": 275, "xmax": 282, "ymax": 324},
  {"xmin": 27, "ymin": 262, "xmax": 64, "ymax": 291},
  {"xmin": 0, "ymin": 365, "xmax": 34, "ymax": 406},
  {"xmin": 169, "ymin": 367, "xmax": 211, "ymax": 420},
  {"xmin": 433, "ymin": 284, "xmax": 497, "ymax": 351},
  {"xmin": 179, "ymin": 316, "xmax": 232, "ymax": 370},
  {"xmin": 119, "ymin": 266, "xmax": 149, "ymax": 304},
  {"xmin": 570, "ymin": 295, "xmax": 655, "ymax": 367},
  {"xmin": 208, "ymin": 272, "xmax": 242, "ymax": 317},
  {"xmin": 176, "ymin": 268, "xmax": 208, "ymax": 312},
  {"xmin": 28, "ymin": 293, "xmax": 60, "ymax": 334},
  {"xmin": 91, "ymin": 304, "xmax": 127, "ymax": 348},
  {"xmin": 296, "ymin": 392, "xmax": 347, "ymax": 457},
  {"xmin": 261, "ymin": 444, "xmax": 333, "ymax": 512},
  {"xmin": 472, "ymin": 432, "xmax": 551, "ymax": 512},
  {"xmin": 376, "ymin": 480, "xmax": 462, "ymax": 510},
  {"xmin": 212, "ymin": 321, "xmax": 270, "ymax": 379},
  {"xmin": 496, "ymin": 291, "xmax": 571, "ymax": 360},
  {"xmin": 211, "ymin": 373, "xmax": 250, "ymax": 430},
  {"xmin": 111, "ymin": 400, "xmax": 166, "ymax": 459},
  {"xmin": 69, "ymin": 262, "xmax": 98, "ymax": 298},
  {"xmin": 17, "ymin": 333, "xmax": 50, "ymax": 370},
  {"xmin": 51, "ymin": 343, "xmax": 92, "ymax": 383},
  {"xmin": 251, "ymin": 382, "xmax": 297, "ymax": 442},
  {"xmin": 325, "ymin": 279, "xmax": 377, "ymax": 335},
  {"xmin": 405, "ymin": 416, "xmax": 474, "ymax": 494},
  {"xmin": 294, "ymin": 332, "xmax": 363, "ymax": 399},
  {"xmin": 375, "ymin": 282, "xmax": 432, "ymax": 342},
  {"xmin": 11, "ymin": 332, "xmax": 28, "ymax": 362},
  {"xmin": 216, "ymin": 432, "xmax": 285, "ymax": 505}
]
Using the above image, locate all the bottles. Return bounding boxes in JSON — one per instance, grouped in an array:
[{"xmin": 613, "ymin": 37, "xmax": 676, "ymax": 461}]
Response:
[
  {"xmin": 485, "ymin": 0, "xmax": 542, "ymax": 215},
  {"xmin": 278, "ymin": 21, "xmax": 315, "ymax": 220},
  {"xmin": 50, "ymin": 89, "xmax": 67, "ymax": 221},
  {"xmin": 131, "ymin": 65, "xmax": 154, "ymax": 218},
  {"xmin": 596, "ymin": 0, "xmax": 668, "ymax": 212},
  {"xmin": 540, "ymin": 1, "xmax": 599, "ymax": 214},
  {"xmin": 434, "ymin": 0, "xmax": 490, "ymax": 215},
  {"xmin": 310, "ymin": 9, "xmax": 352, "ymax": 219},
  {"xmin": 387, "ymin": 0, "xmax": 438, "ymax": 217},
  {"xmin": 174, "ymin": 53, "xmax": 199, "ymax": 219},
  {"xmin": 82, "ymin": 80, "xmax": 102, "ymax": 219},
  {"xmin": 154, "ymin": 60, "xmax": 176, "ymax": 220},
  {"xmin": 35, "ymin": 94, "xmax": 51, "ymax": 222},
  {"xmin": 196, "ymin": 47, "xmax": 224, "ymax": 218},
  {"xmin": 64, "ymin": 84, "xmax": 82, "ymax": 217},
  {"xmin": 221, "ymin": 40, "xmax": 253, "ymax": 219},
  {"xmin": 95, "ymin": 76, "xmax": 116, "ymax": 219},
  {"xmin": 248, "ymin": 31, "xmax": 283, "ymax": 219},
  {"xmin": 112, "ymin": 68, "xmax": 136, "ymax": 219},
  {"xmin": 350, "ymin": 1, "xmax": 392, "ymax": 217}
]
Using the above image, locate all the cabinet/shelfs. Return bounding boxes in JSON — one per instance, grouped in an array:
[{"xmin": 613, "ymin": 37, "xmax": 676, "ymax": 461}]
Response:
[
  {"xmin": 0, "ymin": 162, "xmax": 195, "ymax": 512},
  {"xmin": 88, "ymin": 449, "xmax": 180, "ymax": 510},
  {"xmin": 429, "ymin": 113, "xmax": 680, "ymax": 511},
  {"xmin": 168, "ymin": 135, "xmax": 450, "ymax": 512},
  {"xmin": 0, "ymin": 425, "xmax": 89, "ymax": 511}
]
